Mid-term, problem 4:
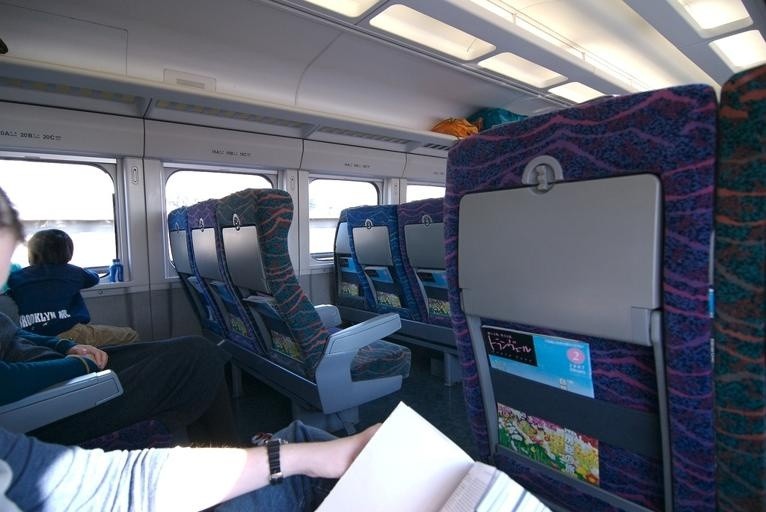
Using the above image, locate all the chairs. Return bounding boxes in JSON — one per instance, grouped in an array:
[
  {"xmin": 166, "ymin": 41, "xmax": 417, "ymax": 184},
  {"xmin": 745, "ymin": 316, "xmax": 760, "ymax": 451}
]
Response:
[
  {"xmin": 397, "ymin": 197, "xmax": 461, "ymax": 389},
  {"xmin": 158, "ymin": 206, "xmax": 241, "ymax": 402},
  {"xmin": 182, "ymin": 195, "xmax": 269, "ymax": 397},
  {"xmin": 441, "ymin": 80, "xmax": 719, "ymax": 510},
  {"xmin": 330, "ymin": 207, "xmax": 370, "ymax": 327},
  {"xmin": 344, "ymin": 201, "xmax": 421, "ymax": 350},
  {"xmin": 215, "ymin": 184, "xmax": 417, "ymax": 436}
]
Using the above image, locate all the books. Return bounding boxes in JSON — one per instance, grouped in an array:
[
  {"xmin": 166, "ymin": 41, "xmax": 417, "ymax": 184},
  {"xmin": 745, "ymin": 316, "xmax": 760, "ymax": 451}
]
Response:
[
  {"xmin": 481, "ymin": 324, "xmax": 602, "ymax": 492},
  {"xmin": 313, "ymin": 400, "xmax": 552, "ymax": 512},
  {"xmin": 185, "ymin": 275, "xmax": 301, "ymax": 361},
  {"xmin": 336, "ymin": 256, "xmax": 450, "ymax": 320}
]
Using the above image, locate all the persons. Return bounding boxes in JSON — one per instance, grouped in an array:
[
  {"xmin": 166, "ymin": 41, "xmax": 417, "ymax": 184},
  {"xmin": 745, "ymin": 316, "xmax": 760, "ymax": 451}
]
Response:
[
  {"xmin": 0, "ymin": 187, "xmax": 388, "ymax": 511},
  {"xmin": 5, "ymin": 227, "xmax": 143, "ymax": 349},
  {"xmin": 0, "ymin": 312, "xmax": 247, "ymax": 450}
]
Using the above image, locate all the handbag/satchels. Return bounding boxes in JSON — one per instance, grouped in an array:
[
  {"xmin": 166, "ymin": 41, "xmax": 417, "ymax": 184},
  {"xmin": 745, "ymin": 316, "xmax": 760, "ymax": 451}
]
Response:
[
  {"xmin": 432, "ymin": 117, "xmax": 478, "ymax": 140},
  {"xmin": 468, "ymin": 108, "xmax": 529, "ymax": 132}
]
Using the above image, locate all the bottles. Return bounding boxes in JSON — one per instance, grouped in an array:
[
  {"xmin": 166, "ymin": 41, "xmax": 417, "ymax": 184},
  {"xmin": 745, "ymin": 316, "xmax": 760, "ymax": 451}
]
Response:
[{"xmin": 110, "ymin": 259, "xmax": 123, "ymax": 282}]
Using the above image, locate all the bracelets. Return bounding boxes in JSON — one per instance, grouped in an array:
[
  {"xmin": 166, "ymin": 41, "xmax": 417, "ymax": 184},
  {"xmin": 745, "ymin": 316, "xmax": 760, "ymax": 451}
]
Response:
[
  {"xmin": 64, "ymin": 353, "xmax": 91, "ymax": 375},
  {"xmin": 262, "ymin": 436, "xmax": 289, "ymax": 487}
]
